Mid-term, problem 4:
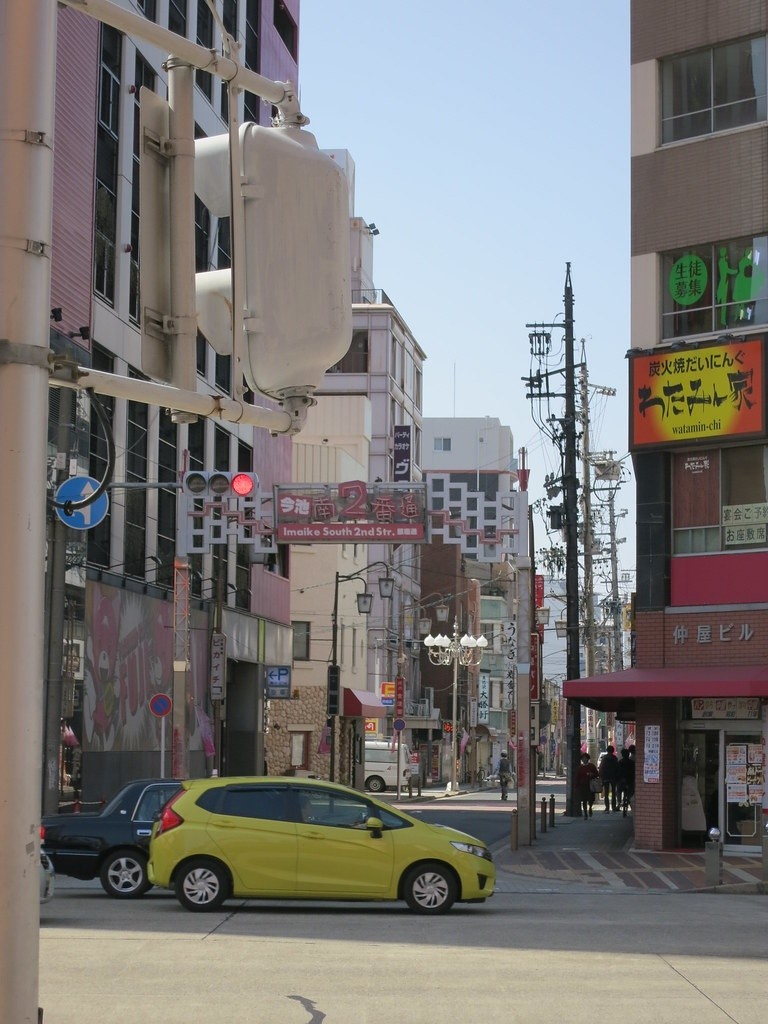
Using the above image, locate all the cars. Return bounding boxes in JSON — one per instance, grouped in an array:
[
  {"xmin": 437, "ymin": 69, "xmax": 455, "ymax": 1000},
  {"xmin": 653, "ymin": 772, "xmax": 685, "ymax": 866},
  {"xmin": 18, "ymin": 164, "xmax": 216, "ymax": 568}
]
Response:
[
  {"xmin": 142, "ymin": 774, "xmax": 497, "ymax": 917},
  {"xmin": 35, "ymin": 778, "xmax": 186, "ymax": 899}
]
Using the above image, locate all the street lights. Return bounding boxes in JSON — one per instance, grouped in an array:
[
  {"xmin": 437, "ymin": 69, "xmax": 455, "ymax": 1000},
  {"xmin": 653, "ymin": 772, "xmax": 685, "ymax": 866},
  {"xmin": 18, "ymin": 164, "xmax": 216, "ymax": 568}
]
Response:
[
  {"xmin": 323, "ymin": 561, "xmax": 394, "ymax": 784},
  {"xmin": 423, "ymin": 614, "xmax": 486, "ymax": 797}
]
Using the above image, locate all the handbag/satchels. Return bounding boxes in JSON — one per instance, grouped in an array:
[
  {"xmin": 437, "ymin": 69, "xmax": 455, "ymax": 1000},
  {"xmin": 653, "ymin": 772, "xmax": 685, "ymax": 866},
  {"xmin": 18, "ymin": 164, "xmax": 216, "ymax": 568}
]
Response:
[{"xmin": 589, "ymin": 768, "xmax": 604, "ymax": 793}]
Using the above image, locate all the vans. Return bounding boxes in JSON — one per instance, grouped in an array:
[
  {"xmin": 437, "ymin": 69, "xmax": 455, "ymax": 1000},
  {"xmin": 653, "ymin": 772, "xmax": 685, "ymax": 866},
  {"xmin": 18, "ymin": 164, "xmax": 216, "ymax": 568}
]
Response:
[{"xmin": 363, "ymin": 739, "xmax": 411, "ymax": 792}]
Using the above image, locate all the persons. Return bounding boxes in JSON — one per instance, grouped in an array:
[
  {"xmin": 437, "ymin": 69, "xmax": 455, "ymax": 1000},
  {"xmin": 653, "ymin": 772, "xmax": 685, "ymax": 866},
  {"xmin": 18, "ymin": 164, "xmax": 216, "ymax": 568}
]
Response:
[
  {"xmin": 615, "ymin": 748, "xmax": 636, "ymax": 811},
  {"xmin": 629, "ymin": 745, "xmax": 635, "ymax": 760},
  {"xmin": 498, "ymin": 750, "xmax": 512, "ymax": 802},
  {"xmin": 599, "ymin": 745, "xmax": 620, "ymax": 811},
  {"xmin": 574, "ymin": 753, "xmax": 598, "ymax": 819}
]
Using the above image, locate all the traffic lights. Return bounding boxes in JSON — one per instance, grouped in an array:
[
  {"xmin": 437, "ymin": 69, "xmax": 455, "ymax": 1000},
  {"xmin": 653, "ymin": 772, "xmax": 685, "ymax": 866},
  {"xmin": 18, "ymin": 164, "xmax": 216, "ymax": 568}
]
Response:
[{"xmin": 183, "ymin": 470, "xmax": 259, "ymax": 499}]
